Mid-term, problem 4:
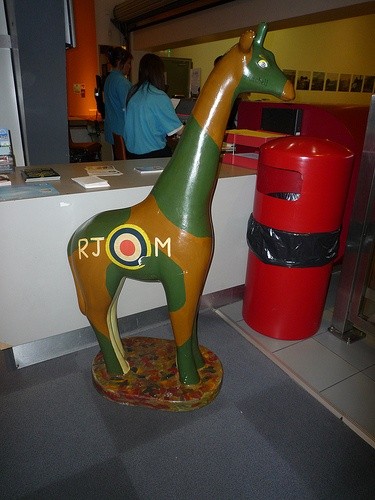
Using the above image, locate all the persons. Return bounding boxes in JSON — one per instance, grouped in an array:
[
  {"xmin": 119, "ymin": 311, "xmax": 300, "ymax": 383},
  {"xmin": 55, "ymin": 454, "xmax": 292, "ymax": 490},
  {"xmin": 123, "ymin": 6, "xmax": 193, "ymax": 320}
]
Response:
[
  {"xmin": 123, "ymin": 53, "xmax": 185, "ymax": 160},
  {"xmin": 214, "ymin": 55, "xmax": 242, "ymax": 130},
  {"xmin": 103, "ymin": 46, "xmax": 134, "ymax": 161}
]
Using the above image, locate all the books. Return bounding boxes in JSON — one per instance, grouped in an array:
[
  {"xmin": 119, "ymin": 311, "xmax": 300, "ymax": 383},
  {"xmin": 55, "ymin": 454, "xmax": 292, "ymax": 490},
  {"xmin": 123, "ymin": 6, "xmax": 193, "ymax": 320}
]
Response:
[{"xmin": 0, "ymin": 128, "xmax": 164, "ymax": 189}]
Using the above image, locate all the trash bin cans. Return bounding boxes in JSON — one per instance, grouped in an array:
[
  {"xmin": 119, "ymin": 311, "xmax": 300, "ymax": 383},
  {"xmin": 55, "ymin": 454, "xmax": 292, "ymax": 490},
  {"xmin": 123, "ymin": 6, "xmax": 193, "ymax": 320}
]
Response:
[{"xmin": 242, "ymin": 135, "xmax": 354, "ymax": 341}]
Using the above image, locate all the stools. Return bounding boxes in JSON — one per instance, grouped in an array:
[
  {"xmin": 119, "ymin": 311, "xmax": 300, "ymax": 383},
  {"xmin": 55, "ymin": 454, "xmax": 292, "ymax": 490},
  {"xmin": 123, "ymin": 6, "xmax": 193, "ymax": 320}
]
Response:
[
  {"xmin": 70, "ymin": 141, "xmax": 103, "ymax": 162},
  {"xmin": 112, "ymin": 132, "xmax": 126, "ymax": 161}
]
